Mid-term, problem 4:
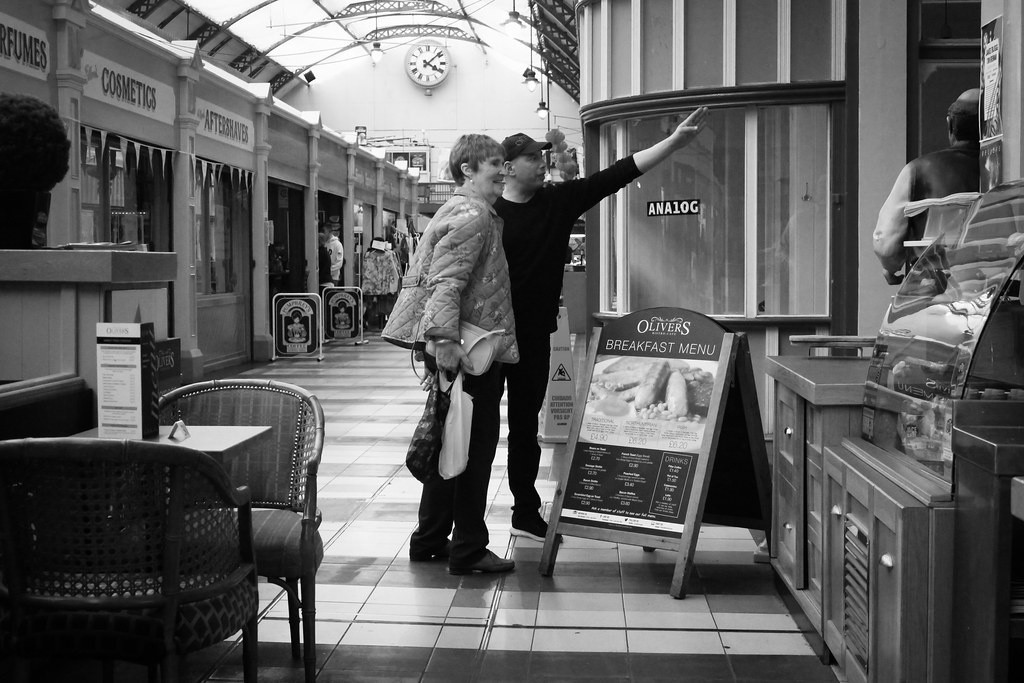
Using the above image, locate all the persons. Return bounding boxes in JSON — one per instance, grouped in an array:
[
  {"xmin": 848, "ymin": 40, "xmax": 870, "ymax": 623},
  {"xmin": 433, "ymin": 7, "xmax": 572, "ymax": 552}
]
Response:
[
  {"xmin": 490, "ymin": 106, "xmax": 712, "ymax": 543},
  {"xmin": 318, "ymin": 223, "xmax": 344, "ymax": 343},
  {"xmin": 380, "ymin": 134, "xmax": 519, "ymax": 576},
  {"xmin": 873, "ymin": 89, "xmax": 981, "ymax": 286}
]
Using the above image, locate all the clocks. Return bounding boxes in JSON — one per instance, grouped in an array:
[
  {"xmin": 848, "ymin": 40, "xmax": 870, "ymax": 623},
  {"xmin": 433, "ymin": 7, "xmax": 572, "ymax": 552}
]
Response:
[{"xmin": 405, "ymin": 38, "xmax": 451, "ymax": 87}]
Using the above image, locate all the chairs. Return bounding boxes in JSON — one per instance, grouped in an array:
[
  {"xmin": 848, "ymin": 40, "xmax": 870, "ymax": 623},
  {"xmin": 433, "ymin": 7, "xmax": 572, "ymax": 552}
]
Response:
[
  {"xmin": 160, "ymin": 377, "xmax": 323, "ymax": 683},
  {"xmin": 0, "ymin": 438, "xmax": 259, "ymax": 683}
]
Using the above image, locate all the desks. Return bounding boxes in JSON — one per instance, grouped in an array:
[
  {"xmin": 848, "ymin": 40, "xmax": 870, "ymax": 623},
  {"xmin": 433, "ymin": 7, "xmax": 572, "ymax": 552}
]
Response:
[{"xmin": 57, "ymin": 424, "xmax": 274, "ymax": 683}]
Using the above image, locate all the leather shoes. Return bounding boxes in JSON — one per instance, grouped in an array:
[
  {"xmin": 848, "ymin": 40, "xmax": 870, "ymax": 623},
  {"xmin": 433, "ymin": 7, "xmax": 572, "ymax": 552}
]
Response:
[
  {"xmin": 410, "ymin": 539, "xmax": 453, "ymax": 562},
  {"xmin": 449, "ymin": 549, "xmax": 515, "ymax": 576}
]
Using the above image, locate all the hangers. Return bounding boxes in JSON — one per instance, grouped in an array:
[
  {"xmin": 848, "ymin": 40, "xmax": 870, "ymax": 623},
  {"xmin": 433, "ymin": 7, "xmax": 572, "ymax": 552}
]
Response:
[{"xmin": 372, "ymin": 248, "xmax": 388, "ymax": 254}]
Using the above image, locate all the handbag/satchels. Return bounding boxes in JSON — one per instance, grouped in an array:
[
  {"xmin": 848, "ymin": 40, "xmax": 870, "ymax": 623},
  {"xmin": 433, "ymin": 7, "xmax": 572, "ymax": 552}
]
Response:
[
  {"xmin": 406, "ymin": 369, "xmax": 473, "ymax": 484},
  {"xmin": 426, "ymin": 318, "xmax": 506, "ymax": 376}
]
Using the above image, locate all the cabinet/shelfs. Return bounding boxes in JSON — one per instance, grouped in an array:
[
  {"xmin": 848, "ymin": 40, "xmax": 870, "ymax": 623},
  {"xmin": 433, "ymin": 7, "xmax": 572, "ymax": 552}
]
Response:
[{"xmin": 770, "ymin": 378, "xmax": 864, "ymax": 606}]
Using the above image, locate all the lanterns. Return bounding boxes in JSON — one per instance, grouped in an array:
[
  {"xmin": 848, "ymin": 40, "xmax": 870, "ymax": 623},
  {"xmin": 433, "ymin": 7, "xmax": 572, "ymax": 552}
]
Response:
[{"xmin": 544, "ymin": 127, "xmax": 578, "ymax": 181}]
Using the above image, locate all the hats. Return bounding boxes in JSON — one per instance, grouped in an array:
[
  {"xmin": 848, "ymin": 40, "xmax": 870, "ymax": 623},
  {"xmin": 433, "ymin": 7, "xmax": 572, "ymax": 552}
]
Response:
[{"xmin": 501, "ymin": 133, "xmax": 553, "ymax": 163}]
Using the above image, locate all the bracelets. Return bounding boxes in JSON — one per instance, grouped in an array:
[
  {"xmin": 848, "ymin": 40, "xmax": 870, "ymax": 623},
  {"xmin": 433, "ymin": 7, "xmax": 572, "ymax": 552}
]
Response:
[{"xmin": 434, "ymin": 339, "xmax": 455, "ymax": 344}]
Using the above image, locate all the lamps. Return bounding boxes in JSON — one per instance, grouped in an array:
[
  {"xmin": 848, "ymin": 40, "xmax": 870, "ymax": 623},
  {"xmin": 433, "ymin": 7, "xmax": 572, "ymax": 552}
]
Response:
[
  {"xmin": 521, "ymin": 0, "xmax": 541, "ymax": 90},
  {"xmin": 535, "ymin": 50, "xmax": 551, "ymax": 119},
  {"xmin": 304, "ymin": 71, "xmax": 316, "ymax": 83},
  {"xmin": 370, "ymin": 17, "xmax": 385, "ymax": 62},
  {"xmin": 500, "ymin": 0, "xmax": 526, "ymax": 33}
]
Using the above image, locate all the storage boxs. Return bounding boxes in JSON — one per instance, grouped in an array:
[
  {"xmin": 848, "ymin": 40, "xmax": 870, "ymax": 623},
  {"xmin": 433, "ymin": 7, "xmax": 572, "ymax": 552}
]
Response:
[{"xmin": 94, "ymin": 320, "xmax": 158, "ymax": 442}]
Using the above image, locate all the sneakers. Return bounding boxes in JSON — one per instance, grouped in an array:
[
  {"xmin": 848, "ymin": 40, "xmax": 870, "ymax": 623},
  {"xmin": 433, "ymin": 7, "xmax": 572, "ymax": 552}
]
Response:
[{"xmin": 509, "ymin": 505, "xmax": 563, "ymax": 543}]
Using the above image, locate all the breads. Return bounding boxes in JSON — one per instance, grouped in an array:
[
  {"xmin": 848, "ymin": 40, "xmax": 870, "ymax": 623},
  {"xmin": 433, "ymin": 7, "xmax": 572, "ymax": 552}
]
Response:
[{"xmin": 591, "ymin": 356, "xmax": 713, "ymax": 419}]
